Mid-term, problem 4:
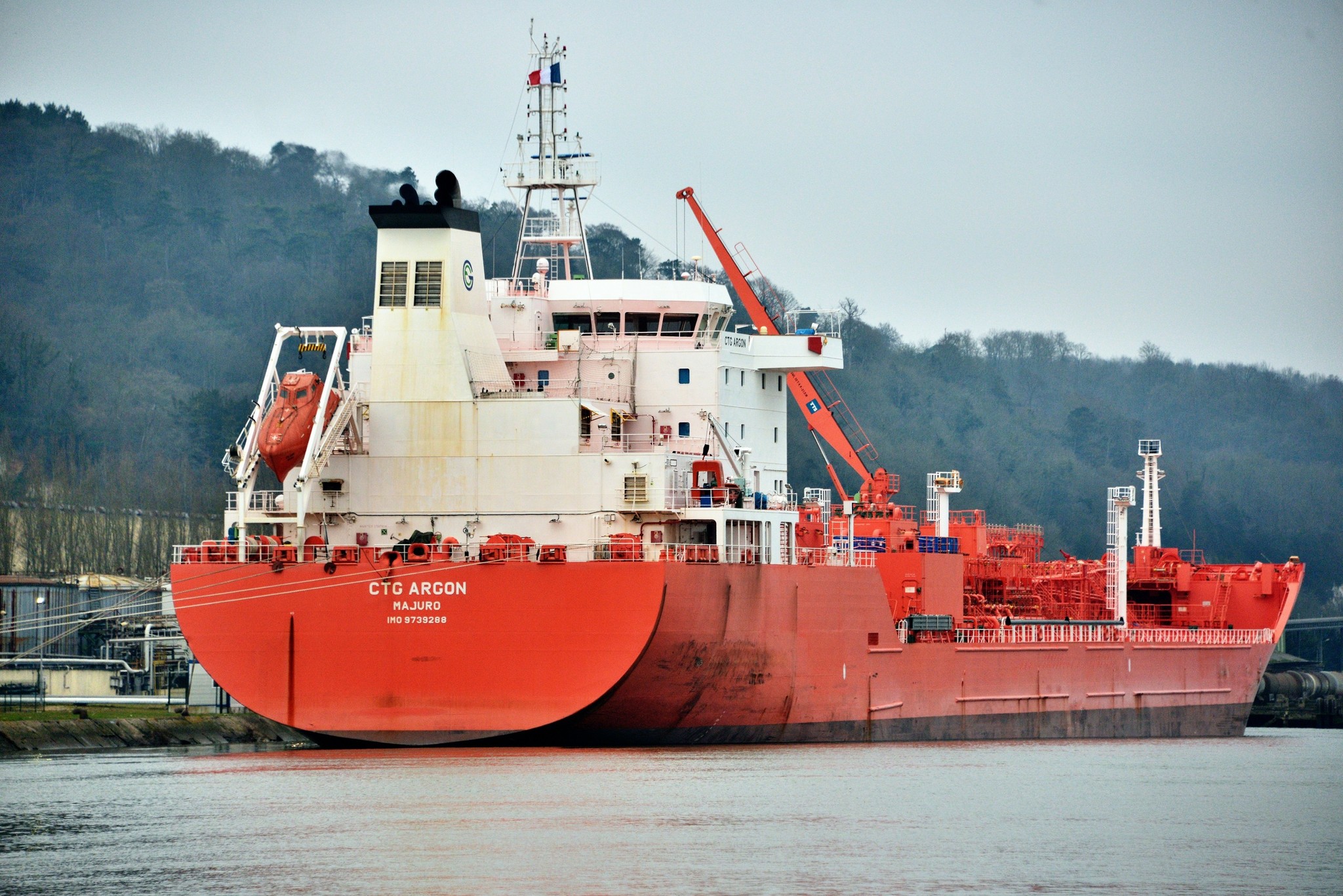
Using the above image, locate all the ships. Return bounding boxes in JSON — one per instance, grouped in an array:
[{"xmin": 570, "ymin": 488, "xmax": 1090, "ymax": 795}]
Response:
[{"xmin": 169, "ymin": 17, "xmax": 1305, "ymax": 746}]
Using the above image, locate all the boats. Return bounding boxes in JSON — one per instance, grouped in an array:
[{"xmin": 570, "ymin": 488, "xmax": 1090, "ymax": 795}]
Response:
[{"xmin": 257, "ymin": 369, "xmax": 342, "ymax": 482}]
